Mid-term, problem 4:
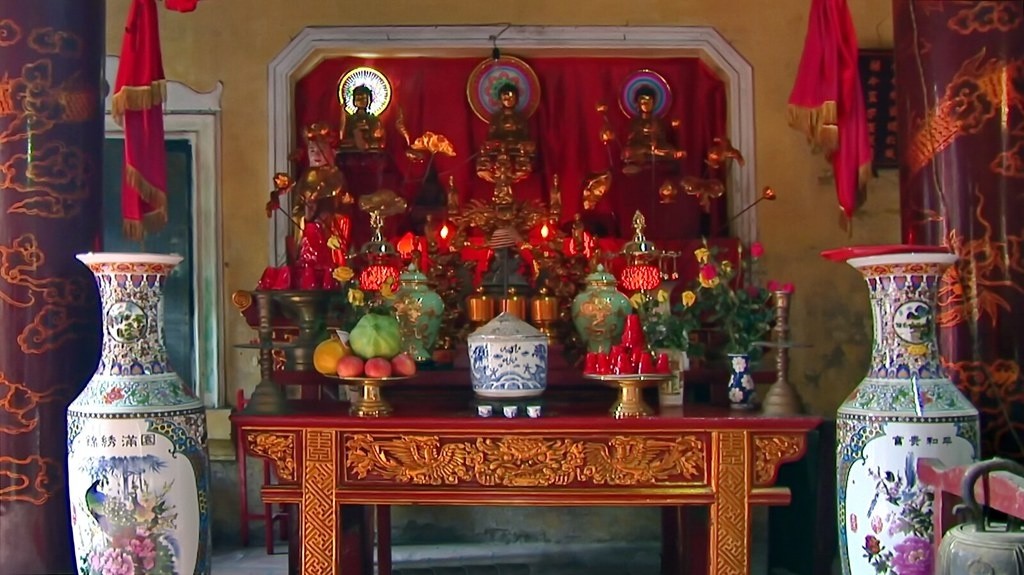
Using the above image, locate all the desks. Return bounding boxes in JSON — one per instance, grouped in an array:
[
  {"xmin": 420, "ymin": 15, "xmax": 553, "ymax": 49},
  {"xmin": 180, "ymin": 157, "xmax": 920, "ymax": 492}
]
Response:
[{"xmin": 228, "ymin": 393, "xmax": 823, "ymax": 575}]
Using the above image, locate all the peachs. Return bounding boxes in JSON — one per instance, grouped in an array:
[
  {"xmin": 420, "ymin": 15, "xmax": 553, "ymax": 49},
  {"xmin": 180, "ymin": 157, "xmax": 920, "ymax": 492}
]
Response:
[{"xmin": 337, "ymin": 353, "xmax": 416, "ymax": 378}]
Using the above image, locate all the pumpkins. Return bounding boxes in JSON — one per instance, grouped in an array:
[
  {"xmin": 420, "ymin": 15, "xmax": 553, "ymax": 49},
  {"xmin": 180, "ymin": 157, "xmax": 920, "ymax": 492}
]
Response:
[{"xmin": 313, "ymin": 328, "xmax": 353, "ymax": 374}]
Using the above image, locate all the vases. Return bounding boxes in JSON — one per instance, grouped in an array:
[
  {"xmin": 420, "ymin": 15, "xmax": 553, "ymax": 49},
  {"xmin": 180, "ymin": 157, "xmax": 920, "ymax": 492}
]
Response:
[
  {"xmin": 836, "ymin": 253, "xmax": 979, "ymax": 575},
  {"xmin": 68, "ymin": 249, "xmax": 214, "ymax": 575}
]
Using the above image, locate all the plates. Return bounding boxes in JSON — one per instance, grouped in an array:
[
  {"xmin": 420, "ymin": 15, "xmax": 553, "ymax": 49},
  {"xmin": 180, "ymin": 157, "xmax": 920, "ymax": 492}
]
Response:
[{"xmin": 819, "ymin": 245, "xmax": 950, "ymax": 264}]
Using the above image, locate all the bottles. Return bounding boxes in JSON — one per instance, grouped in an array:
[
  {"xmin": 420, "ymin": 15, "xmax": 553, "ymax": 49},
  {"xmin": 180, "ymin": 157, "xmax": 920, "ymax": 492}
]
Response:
[
  {"xmin": 835, "ymin": 252, "xmax": 980, "ymax": 574},
  {"xmin": 65, "ymin": 251, "xmax": 212, "ymax": 575}
]
